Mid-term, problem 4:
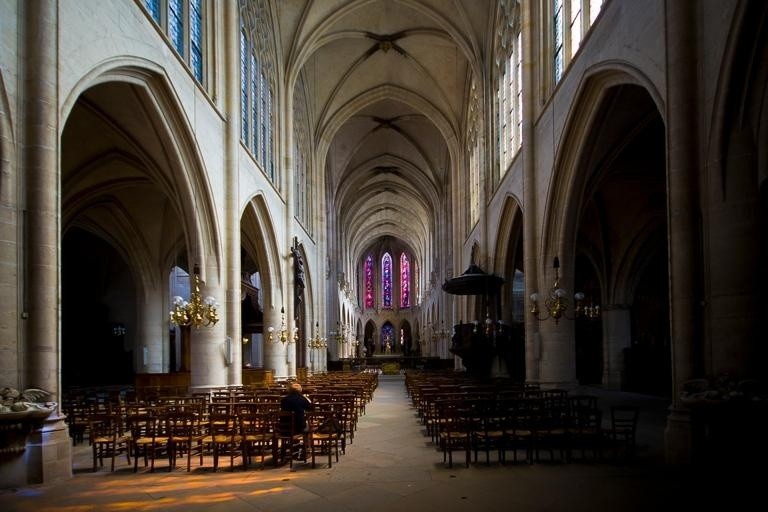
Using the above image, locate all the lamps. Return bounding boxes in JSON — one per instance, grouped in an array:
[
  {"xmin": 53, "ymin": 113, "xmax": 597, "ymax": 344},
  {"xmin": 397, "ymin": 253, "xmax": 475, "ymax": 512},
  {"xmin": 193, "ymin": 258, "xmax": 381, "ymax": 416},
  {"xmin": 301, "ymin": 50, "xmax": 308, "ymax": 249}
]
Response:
[
  {"xmin": 413, "ymin": 278, "xmax": 516, "ymax": 347},
  {"xmin": 163, "ymin": 1, "xmax": 223, "ymax": 333},
  {"xmin": 263, "ymin": 304, "xmax": 363, "ymax": 351},
  {"xmin": 528, "ymin": 1, "xmax": 603, "ymax": 327}
]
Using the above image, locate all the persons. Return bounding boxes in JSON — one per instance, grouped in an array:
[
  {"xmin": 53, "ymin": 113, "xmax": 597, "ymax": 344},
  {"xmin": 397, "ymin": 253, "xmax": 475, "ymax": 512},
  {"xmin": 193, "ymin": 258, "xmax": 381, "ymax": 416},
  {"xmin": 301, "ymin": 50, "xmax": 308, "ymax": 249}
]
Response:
[{"xmin": 280, "ymin": 383, "xmax": 312, "ymax": 459}]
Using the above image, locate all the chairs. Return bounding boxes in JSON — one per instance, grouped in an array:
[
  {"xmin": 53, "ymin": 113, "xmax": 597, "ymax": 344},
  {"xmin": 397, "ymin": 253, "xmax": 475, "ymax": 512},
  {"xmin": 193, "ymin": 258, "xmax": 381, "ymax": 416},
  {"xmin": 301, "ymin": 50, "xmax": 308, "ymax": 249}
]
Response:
[
  {"xmin": 61, "ymin": 368, "xmax": 380, "ymax": 475},
  {"xmin": 403, "ymin": 368, "xmax": 647, "ymax": 469}
]
